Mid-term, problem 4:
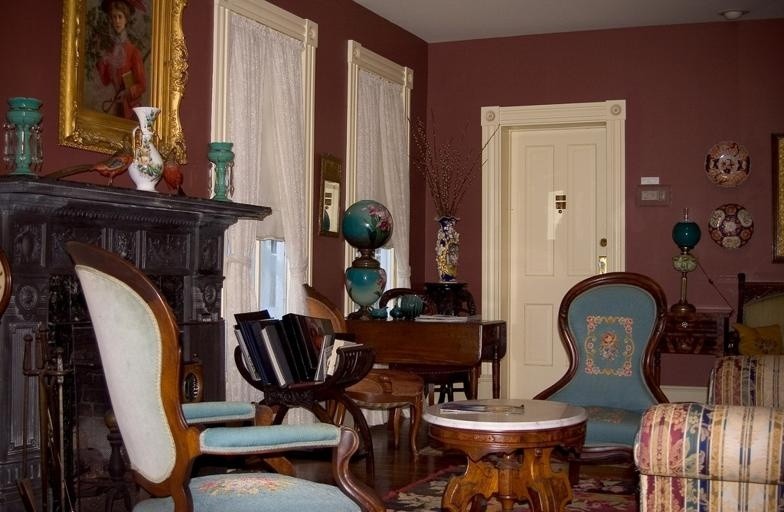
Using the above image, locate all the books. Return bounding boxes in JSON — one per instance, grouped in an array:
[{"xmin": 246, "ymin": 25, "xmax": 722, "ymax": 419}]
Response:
[
  {"xmin": 415, "ymin": 313, "xmax": 482, "ymax": 323},
  {"xmin": 234, "ymin": 310, "xmax": 364, "ymax": 389}
]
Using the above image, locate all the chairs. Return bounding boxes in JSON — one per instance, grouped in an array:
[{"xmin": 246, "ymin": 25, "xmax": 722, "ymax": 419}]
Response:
[
  {"xmin": 297, "ymin": 278, "xmax": 427, "ymax": 463},
  {"xmin": 524, "ymin": 268, "xmax": 672, "ymax": 493},
  {"xmin": 60, "ymin": 230, "xmax": 390, "ymax": 512},
  {"xmin": 378, "ymin": 284, "xmax": 479, "ymax": 406}
]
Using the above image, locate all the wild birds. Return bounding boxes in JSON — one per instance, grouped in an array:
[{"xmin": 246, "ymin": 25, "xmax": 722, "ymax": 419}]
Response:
[
  {"xmin": 44, "ymin": 133, "xmax": 134, "ymax": 186},
  {"xmin": 163, "ymin": 146, "xmax": 188, "ymax": 196}
]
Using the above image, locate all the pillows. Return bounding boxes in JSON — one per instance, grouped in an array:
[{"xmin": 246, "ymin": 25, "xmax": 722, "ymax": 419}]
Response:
[{"xmin": 732, "ymin": 318, "xmax": 783, "ymax": 355}]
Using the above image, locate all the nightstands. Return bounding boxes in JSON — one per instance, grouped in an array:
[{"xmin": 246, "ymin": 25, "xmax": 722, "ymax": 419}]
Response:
[{"xmin": 646, "ymin": 305, "xmax": 734, "ymax": 387}]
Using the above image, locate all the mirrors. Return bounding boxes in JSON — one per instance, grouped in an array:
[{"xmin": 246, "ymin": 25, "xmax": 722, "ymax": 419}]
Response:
[{"xmin": 317, "ymin": 150, "xmax": 345, "ymax": 240}]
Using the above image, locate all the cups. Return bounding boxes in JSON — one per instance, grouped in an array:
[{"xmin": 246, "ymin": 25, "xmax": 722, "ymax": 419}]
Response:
[
  {"xmin": 395, "ymin": 294, "xmax": 422, "ymax": 319},
  {"xmin": 367, "ymin": 306, "xmax": 388, "ymax": 319}
]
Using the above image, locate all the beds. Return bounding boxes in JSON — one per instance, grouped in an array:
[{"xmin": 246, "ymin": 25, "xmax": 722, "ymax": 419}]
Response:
[{"xmin": 726, "ymin": 269, "xmax": 783, "ymax": 360}]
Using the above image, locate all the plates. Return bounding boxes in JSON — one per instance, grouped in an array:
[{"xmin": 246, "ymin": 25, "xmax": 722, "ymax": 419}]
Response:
[
  {"xmin": 704, "ymin": 142, "xmax": 751, "ymax": 185},
  {"xmin": 707, "ymin": 205, "xmax": 755, "ymax": 250}
]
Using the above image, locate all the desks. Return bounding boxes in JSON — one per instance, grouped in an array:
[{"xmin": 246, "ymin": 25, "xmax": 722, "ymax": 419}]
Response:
[
  {"xmin": 343, "ymin": 310, "xmax": 509, "ymax": 401},
  {"xmin": 419, "ymin": 392, "xmax": 590, "ymax": 512}
]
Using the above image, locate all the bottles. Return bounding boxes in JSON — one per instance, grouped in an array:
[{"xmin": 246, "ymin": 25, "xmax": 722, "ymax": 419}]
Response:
[
  {"xmin": 390, "ymin": 305, "xmax": 402, "ymax": 319},
  {"xmin": 128, "ymin": 108, "xmax": 163, "ymax": 192}
]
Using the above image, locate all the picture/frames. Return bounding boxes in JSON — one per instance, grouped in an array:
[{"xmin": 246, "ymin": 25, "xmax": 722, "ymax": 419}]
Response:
[
  {"xmin": 58, "ymin": 0, "xmax": 190, "ymax": 167},
  {"xmin": 767, "ymin": 128, "xmax": 783, "ymax": 264}
]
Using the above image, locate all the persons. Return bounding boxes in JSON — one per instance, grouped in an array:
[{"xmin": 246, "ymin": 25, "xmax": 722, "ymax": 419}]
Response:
[{"xmin": 93, "ymin": 0, "xmax": 146, "ymax": 121}]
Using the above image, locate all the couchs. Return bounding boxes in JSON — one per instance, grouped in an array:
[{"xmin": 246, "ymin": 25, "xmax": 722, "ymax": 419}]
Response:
[{"xmin": 626, "ymin": 347, "xmax": 784, "ymax": 512}]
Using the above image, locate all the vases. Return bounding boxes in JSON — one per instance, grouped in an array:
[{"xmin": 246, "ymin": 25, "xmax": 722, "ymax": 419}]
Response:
[
  {"xmin": 129, "ymin": 103, "xmax": 168, "ymax": 193},
  {"xmin": 204, "ymin": 139, "xmax": 238, "ymax": 204},
  {"xmin": 434, "ymin": 215, "xmax": 463, "ymax": 284},
  {"xmin": 2, "ymin": 92, "xmax": 49, "ymax": 183}
]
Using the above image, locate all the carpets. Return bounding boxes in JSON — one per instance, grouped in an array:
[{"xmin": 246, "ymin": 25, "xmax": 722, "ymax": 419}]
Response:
[{"xmin": 375, "ymin": 461, "xmax": 641, "ymax": 512}]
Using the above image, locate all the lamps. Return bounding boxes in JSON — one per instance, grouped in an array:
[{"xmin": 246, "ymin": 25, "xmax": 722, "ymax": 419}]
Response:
[{"xmin": 668, "ymin": 204, "xmax": 703, "ymax": 311}]
